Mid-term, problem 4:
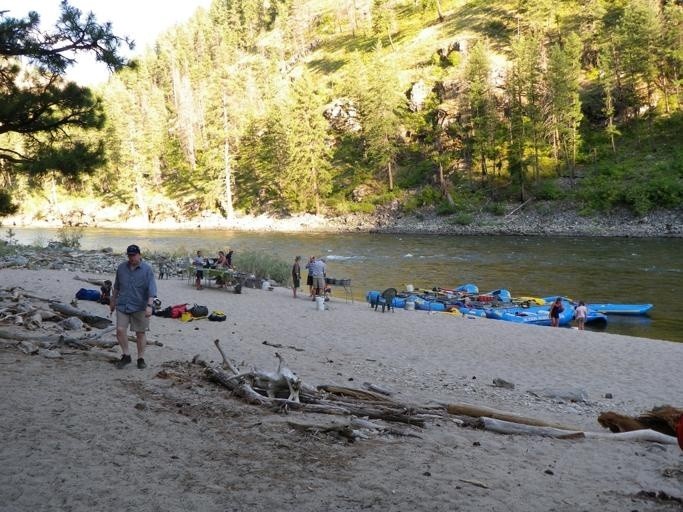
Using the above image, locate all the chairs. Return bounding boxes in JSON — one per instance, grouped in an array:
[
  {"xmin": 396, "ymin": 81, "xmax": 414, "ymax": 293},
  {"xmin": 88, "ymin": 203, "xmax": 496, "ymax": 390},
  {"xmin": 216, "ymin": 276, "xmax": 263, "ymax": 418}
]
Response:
[{"xmin": 374, "ymin": 287, "xmax": 397, "ymax": 313}]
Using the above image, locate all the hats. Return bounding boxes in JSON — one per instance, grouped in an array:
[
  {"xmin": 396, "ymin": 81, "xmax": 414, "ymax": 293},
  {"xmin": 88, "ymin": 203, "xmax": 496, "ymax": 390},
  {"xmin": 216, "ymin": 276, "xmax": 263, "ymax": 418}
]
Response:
[{"xmin": 125, "ymin": 245, "xmax": 140, "ymax": 255}]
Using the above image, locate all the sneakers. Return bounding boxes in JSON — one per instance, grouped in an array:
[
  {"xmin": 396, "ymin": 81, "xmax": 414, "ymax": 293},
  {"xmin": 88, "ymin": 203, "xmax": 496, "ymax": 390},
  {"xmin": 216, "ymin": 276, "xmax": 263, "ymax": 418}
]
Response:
[
  {"xmin": 137, "ymin": 358, "xmax": 146, "ymax": 368},
  {"xmin": 117, "ymin": 354, "xmax": 131, "ymax": 368}
]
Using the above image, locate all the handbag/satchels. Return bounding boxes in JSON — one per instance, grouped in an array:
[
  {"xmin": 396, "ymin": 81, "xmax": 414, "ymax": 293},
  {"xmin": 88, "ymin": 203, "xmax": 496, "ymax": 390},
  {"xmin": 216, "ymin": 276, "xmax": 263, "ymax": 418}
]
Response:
[
  {"xmin": 208, "ymin": 310, "xmax": 226, "ymax": 321},
  {"xmin": 76, "ymin": 288, "xmax": 100, "ymax": 301},
  {"xmin": 156, "ymin": 303, "xmax": 208, "ymax": 319}
]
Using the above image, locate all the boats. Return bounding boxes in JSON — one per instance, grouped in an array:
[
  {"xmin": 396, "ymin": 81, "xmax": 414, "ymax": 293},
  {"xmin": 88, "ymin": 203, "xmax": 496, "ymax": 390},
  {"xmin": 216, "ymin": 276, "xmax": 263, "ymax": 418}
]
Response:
[{"xmin": 367, "ymin": 283, "xmax": 657, "ymax": 329}]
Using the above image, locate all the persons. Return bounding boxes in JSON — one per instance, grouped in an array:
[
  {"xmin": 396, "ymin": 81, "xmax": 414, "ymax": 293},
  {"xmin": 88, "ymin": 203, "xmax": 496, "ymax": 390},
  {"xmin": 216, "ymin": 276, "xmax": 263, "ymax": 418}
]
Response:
[
  {"xmin": 212, "ymin": 250, "xmax": 227, "ymax": 289},
  {"xmin": 304, "ymin": 255, "xmax": 317, "ymax": 298},
  {"xmin": 225, "ymin": 249, "xmax": 233, "ymax": 286},
  {"xmin": 574, "ymin": 300, "xmax": 588, "ymax": 330},
  {"xmin": 549, "ymin": 296, "xmax": 563, "ymax": 328},
  {"xmin": 194, "ymin": 251, "xmax": 203, "ymax": 290},
  {"xmin": 310, "ymin": 258, "xmax": 330, "ymax": 301},
  {"xmin": 107, "ymin": 244, "xmax": 157, "ymax": 372},
  {"xmin": 290, "ymin": 255, "xmax": 301, "ymax": 298}
]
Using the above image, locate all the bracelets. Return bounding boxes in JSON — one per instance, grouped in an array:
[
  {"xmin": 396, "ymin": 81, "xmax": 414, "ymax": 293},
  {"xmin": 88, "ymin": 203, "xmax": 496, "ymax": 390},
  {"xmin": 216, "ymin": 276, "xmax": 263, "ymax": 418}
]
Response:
[{"xmin": 145, "ymin": 303, "xmax": 152, "ymax": 308}]
